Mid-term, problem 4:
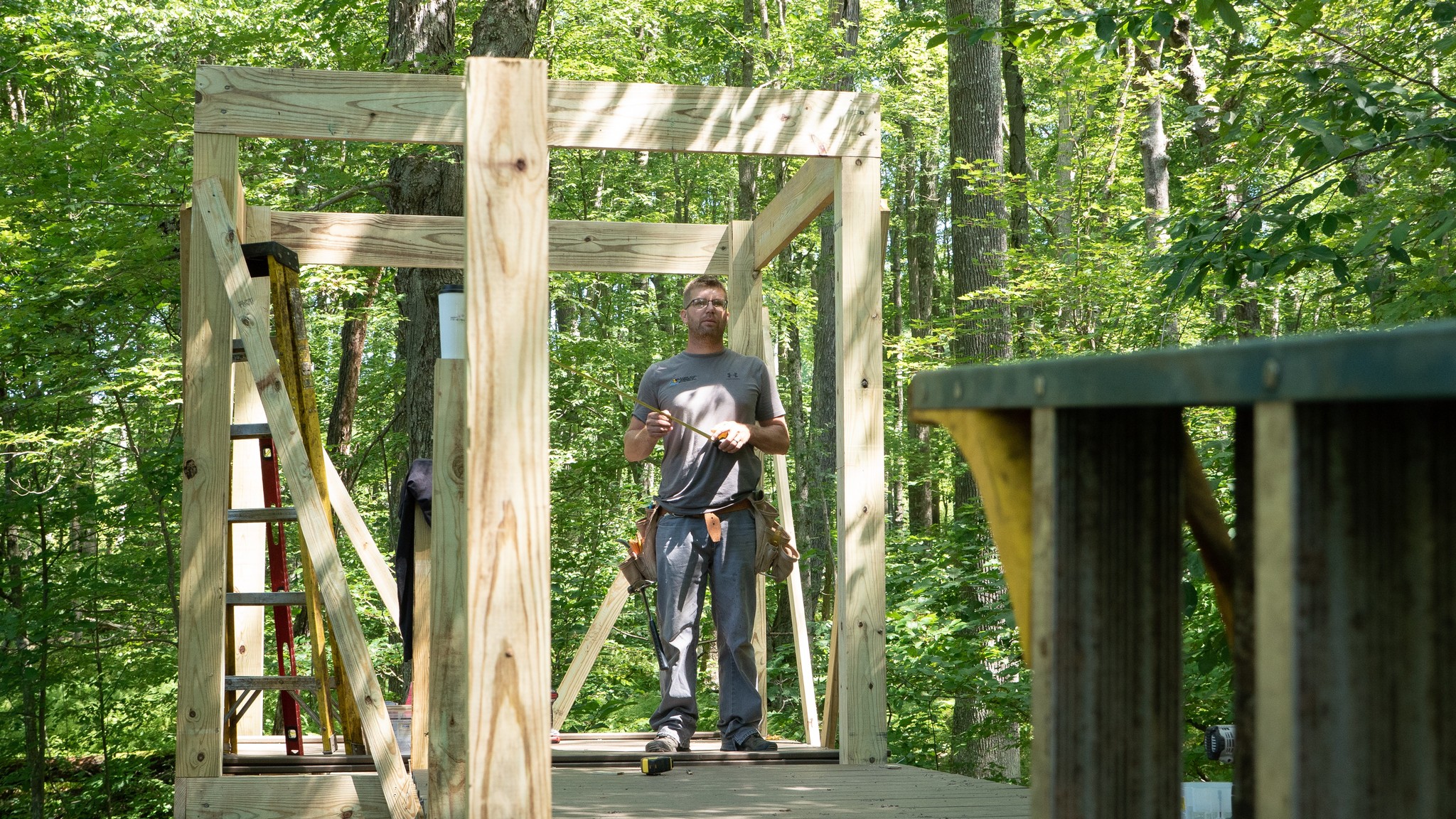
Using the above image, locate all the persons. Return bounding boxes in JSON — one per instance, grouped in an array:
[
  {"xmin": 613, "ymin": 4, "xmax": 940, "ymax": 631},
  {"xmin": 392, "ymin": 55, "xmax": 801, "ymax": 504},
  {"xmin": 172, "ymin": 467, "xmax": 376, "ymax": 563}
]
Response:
[{"xmin": 621, "ymin": 272, "xmax": 790, "ymax": 752}]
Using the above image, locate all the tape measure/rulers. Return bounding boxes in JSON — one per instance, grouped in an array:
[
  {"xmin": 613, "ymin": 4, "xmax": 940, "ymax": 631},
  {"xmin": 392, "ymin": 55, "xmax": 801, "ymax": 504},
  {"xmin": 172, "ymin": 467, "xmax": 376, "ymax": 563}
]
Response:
[
  {"xmin": 641, "ymin": 757, "xmax": 674, "ymax": 774},
  {"xmin": 548, "ymin": 357, "xmax": 729, "ymax": 447}
]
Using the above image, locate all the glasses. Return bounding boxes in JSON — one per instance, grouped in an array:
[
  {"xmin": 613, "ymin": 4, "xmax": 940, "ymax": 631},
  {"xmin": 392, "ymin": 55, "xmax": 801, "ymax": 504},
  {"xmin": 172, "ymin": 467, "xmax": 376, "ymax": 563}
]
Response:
[{"xmin": 685, "ymin": 298, "xmax": 728, "ymax": 310}]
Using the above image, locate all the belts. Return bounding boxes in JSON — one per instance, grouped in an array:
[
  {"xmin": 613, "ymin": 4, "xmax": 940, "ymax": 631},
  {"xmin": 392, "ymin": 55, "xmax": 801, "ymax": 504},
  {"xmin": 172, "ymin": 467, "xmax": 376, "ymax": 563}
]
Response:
[{"xmin": 659, "ymin": 498, "xmax": 752, "ymax": 542}]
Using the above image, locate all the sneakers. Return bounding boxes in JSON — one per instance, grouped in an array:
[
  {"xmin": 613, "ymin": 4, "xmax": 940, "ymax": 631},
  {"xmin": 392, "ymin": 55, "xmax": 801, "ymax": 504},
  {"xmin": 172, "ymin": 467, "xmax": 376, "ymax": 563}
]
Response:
[
  {"xmin": 645, "ymin": 733, "xmax": 685, "ymax": 753},
  {"xmin": 720, "ymin": 734, "xmax": 778, "ymax": 751}
]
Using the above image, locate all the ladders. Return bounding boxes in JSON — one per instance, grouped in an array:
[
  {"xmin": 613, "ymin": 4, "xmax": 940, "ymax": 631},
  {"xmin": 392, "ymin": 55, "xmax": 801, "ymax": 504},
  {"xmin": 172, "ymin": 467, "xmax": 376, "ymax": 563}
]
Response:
[{"xmin": 222, "ymin": 241, "xmax": 366, "ymax": 757}]
